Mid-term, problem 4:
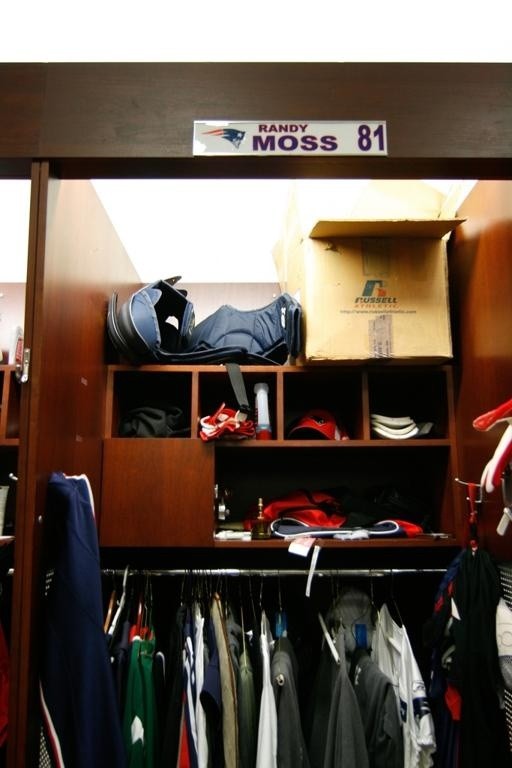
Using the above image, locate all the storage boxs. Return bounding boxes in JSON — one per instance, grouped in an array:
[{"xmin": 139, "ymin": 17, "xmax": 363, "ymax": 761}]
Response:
[{"xmin": 268, "ymin": 218, "xmax": 469, "ymax": 360}]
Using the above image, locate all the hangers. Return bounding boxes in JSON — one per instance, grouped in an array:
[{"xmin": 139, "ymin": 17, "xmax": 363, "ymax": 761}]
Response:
[{"xmin": 473, "ymin": 393, "xmax": 511, "ymax": 539}]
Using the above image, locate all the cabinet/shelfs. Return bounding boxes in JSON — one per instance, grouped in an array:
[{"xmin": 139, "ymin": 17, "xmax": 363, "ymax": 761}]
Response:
[
  {"xmin": 97, "ymin": 361, "xmax": 467, "ymax": 551},
  {"xmin": 1, "ymin": 362, "xmax": 27, "ymax": 541}
]
